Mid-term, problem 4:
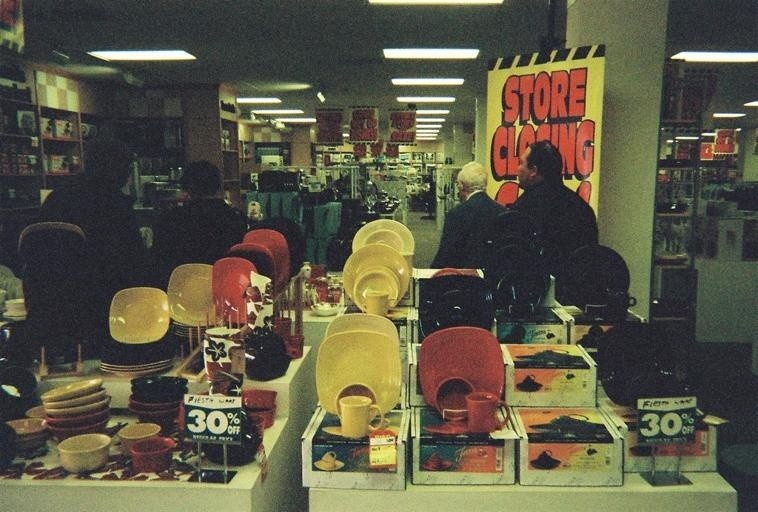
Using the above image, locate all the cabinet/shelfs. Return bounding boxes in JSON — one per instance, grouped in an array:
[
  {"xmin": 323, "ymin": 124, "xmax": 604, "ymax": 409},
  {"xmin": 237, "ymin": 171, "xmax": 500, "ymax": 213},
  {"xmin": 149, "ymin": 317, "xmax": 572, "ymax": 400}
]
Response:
[
  {"xmin": 314, "ymin": 150, "xmax": 461, "ymax": 230},
  {"xmin": 182, "ymin": 83, "xmax": 240, "ymax": 209},
  {"xmin": 0, "ymin": 53, "xmax": 101, "ymax": 213},
  {"xmin": 650, "ymin": 63, "xmax": 702, "ymax": 338}
]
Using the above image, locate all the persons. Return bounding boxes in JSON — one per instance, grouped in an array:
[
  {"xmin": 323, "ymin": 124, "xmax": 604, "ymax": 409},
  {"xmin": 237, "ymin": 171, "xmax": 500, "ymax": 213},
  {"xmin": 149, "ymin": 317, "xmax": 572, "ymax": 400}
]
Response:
[
  {"xmin": 510, "ymin": 140, "xmax": 599, "ymax": 266},
  {"xmin": 160, "ymin": 160, "xmax": 247, "ymax": 266},
  {"xmin": 22, "ymin": 137, "xmax": 151, "ymax": 303},
  {"xmin": 430, "ymin": 161, "xmax": 506, "ymax": 270}
]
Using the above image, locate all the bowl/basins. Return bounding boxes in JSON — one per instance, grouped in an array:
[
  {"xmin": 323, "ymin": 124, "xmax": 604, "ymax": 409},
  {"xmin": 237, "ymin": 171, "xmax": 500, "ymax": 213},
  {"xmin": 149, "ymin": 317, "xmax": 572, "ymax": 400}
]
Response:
[
  {"xmin": 245, "ymin": 406, "xmax": 276, "ymax": 429},
  {"xmin": 116, "ymin": 424, "xmax": 162, "ymax": 452},
  {"xmin": 6, "ymin": 419, "xmax": 50, "ymax": 450},
  {"xmin": 26, "ymin": 406, "xmax": 47, "ymax": 420},
  {"xmin": 125, "ymin": 376, "xmax": 190, "ymax": 432},
  {"xmin": 39, "ymin": 379, "xmax": 113, "ymax": 441},
  {"xmin": 243, "ymin": 388, "xmax": 279, "ymax": 411},
  {"xmin": 434, "ymin": 377, "xmax": 475, "ymax": 420},
  {"xmin": 56, "ymin": 433, "xmax": 112, "ymax": 470},
  {"xmin": 130, "ymin": 436, "xmax": 174, "ymax": 470},
  {"xmin": 335, "ymin": 382, "xmax": 378, "ymax": 421}
]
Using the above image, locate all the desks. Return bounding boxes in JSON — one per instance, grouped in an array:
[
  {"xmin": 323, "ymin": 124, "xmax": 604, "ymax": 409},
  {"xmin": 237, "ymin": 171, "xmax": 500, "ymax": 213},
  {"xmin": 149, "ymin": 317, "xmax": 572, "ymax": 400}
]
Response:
[
  {"xmin": 0, "ymin": 345, "xmax": 311, "ymax": 512},
  {"xmin": 281, "ymin": 310, "xmax": 341, "ymax": 412},
  {"xmin": 308, "ymin": 469, "xmax": 737, "ymax": 512}
]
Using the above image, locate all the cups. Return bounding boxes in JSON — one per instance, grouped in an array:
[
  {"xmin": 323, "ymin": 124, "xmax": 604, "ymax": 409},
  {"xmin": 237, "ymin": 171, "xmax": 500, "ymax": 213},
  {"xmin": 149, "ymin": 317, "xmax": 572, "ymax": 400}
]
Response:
[
  {"xmin": 425, "ymin": 452, "xmax": 444, "ymax": 469},
  {"xmin": 320, "ymin": 451, "xmax": 337, "ymax": 468},
  {"xmin": 273, "ymin": 317, "xmax": 293, "ymax": 342},
  {"xmin": 523, "ymin": 374, "xmax": 537, "ymax": 385},
  {"xmin": 468, "ymin": 391, "xmax": 511, "ymax": 434},
  {"xmin": 340, "ymin": 395, "xmax": 383, "ymax": 439},
  {"xmin": 536, "ymin": 449, "xmax": 553, "ymax": 466},
  {"xmin": 286, "ymin": 335, "xmax": 304, "ymax": 358},
  {"xmin": 550, "ymin": 413, "xmax": 595, "ymax": 434}
]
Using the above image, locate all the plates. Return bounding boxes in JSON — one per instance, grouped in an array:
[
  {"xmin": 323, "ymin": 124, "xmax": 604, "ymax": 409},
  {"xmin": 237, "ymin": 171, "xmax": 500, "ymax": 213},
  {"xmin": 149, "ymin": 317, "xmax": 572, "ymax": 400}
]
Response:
[
  {"xmin": 244, "ymin": 228, "xmax": 290, "ymax": 292},
  {"xmin": 315, "ymin": 331, "xmax": 401, "ymax": 414},
  {"xmin": 419, "ymin": 231, "xmax": 632, "ymax": 336},
  {"xmin": 322, "ymin": 425, "xmax": 401, "ymax": 438},
  {"xmin": 311, "ymin": 218, "xmax": 414, "ymax": 339},
  {"xmin": 228, "ymin": 244, "xmax": 276, "ymax": 265},
  {"xmin": 314, "ymin": 460, "xmax": 345, "ymax": 471},
  {"xmin": 529, "ymin": 423, "xmax": 607, "ymax": 441},
  {"xmin": 516, "ymin": 355, "xmax": 578, "ymax": 365},
  {"xmin": 212, "ymin": 257, "xmax": 257, "ymax": 324},
  {"xmin": 530, "ymin": 458, "xmax": 560, "ymax": 469},
  {"xmin": 230, "ymin": 248, "xmax": 275, "ymax": 294},
  {"xmin": 255, "ymin": 217, "xmax": 305, "ymax": 278},
  {"xmin": 419, "ymin": 327, "xmax": 504, "ymax": 414},
  {"xmin": 421, "ymin": 461, "xmax": 453, "ymax": 470},
  {"xmin": 595, "ymin": 320, "xmax": 693, "ymax": 402},
  {"xmin": 168, "ymin": 262, "xmax": 214, "ymax": 352},
  {"xmin": 98, "ymin": 287, "xmax": 175, "ymax": 378},
  {"xmin": 516, "ymin": 382, "xmax": 542, "ymax": 392},
  {"xmin": 422, "ymin": 422, "xmax": 467, "ymax": 435}
]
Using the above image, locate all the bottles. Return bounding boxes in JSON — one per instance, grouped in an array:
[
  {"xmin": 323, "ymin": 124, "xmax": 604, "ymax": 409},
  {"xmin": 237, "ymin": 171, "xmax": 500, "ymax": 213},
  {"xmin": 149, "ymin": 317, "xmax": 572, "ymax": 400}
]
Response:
[{"xmin": 166, "ymin": 166, "xmax": 184, "ymax": 188}]
